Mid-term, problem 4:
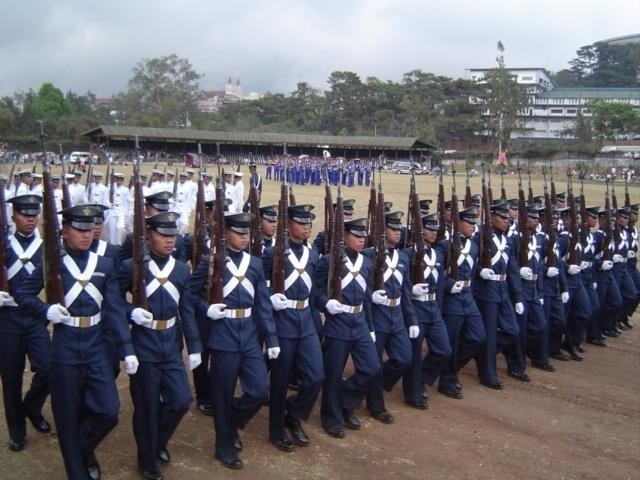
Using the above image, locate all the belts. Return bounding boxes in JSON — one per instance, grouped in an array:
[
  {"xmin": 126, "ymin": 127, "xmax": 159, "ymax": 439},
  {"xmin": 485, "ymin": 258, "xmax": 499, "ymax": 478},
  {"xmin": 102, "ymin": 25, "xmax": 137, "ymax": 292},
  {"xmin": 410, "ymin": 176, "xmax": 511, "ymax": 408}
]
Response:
[
  {"xmin": 150, "ymin": 315, "xmax": 178, "ymax": 330},
  {"xmin": 224, "ymin": 307, "xmax": 254, "ymax": 321},
  {"xmin": 60, "ymin": 311, "xmax": 102, "ymax": 329}
]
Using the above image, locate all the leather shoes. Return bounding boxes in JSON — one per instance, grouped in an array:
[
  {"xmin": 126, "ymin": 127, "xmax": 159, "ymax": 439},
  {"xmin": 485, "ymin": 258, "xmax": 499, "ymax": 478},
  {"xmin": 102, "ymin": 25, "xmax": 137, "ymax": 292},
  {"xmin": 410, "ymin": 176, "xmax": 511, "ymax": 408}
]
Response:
[
  {"xmin": 22, "ymin": 400, "xmax": 51, "ymax": 433},
  {"xmin": 482, "ymin": 320, "xmax": 632, "ymax": 390},
  {"xmin": 200, "ymin": 401, "xmax": 360, "ymax": 469},
  {"xmin": 368, "ymin": 376, "xmax": 464, "ymax": 424},
  {"xmin": 8, "ymin": 431, "xmax": 24, "ymax": 451},
  {"xmin": 73, "ymin": 448, "xmax": 172, "ymax": 479}
]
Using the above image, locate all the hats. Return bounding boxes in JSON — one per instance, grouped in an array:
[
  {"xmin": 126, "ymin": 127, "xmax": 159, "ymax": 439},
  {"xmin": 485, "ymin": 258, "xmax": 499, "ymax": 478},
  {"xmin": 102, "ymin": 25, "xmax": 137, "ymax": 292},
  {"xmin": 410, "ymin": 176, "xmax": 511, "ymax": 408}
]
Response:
[
  {"xmin": 6, "ymin": 192, "xmax": 639, "ymax": 237},
  {"xmin": 0, "ymin": 169, "xmax": 245, "ymax": 184}
]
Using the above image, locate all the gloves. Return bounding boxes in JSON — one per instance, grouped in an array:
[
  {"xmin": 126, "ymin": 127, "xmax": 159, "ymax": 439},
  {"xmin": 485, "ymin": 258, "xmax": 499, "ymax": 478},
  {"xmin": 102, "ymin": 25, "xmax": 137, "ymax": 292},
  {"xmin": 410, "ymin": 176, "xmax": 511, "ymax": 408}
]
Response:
[
  {"xmin": 47, "ymin": 304, "xmax": 69, "ymax": 322},
  {"xmin": 131, "ymin": 308, "xmax": 152, "ymax": 326},
  {"xmin": 127, "ymin": 355, "xmax": 141, "ymax": 377},
  {"xmin": 268, "ymin": 347, "xmax": 281, "ymax": 360},
  {"xmin": 209, "ymin": 304, "xmax": 227, "ymax": 321},
  {"xmin": 460, "ymin": 256, "xmax": 626, "ymax": 288},
  {"xmin": 272, "ymin": 282, "xmax": 465, "ymax": 315},
  {"xmin": 188, "ymin": 352, "xmax": 201, "ymax": 371},
  {"xmin": 371, "ymin": 332, "xmax": 377, "ymax": 344},
  {"xmin": 409, "ymin": 327, "xmax": 420, "ymax": 338},
  {"xmin": 516, "ymin": 282, "xmax": 597, "ymax": 314}
]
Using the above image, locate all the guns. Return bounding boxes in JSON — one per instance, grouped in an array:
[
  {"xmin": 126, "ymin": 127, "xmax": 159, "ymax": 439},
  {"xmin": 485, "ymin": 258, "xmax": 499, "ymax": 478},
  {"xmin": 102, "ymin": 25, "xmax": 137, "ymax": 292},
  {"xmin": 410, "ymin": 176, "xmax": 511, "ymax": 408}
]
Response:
[
  {"xmin": 206, "ymin": 144, "xmax": 226, "ymax": 312},
  {"xmin": 57, "ymin": 143, "xmax": 73, "ymax": 217},
  {"xmin": 130, "ymin": 133, "xmax": 148, "ymax": 316},
  {"xmin": 272, "ymin": 142, "xmax": 289, "ymax": 295},
  {"xmin": 191, "ymin": 143, "xmax": 210, "ymax": 275},
  {"xmin": 367, "ymin": 155, "xmax": 638, "ymax": 301},
  {"xmin": 0, "ymin": 146, "xmax": 363, "ymax": 238},
  {"xmin": 248, "ymin": 152, "xmax": 265, "ymax": 257},
  {"xmin": 323, "ymin": 163, "xmax": 336, "ymax": 258},
  {"xmin": 1, "ymin": 175, "xmax": 11, "ymax": 293},
  {"xmin": 289, "ymin": 184, "xmax": 296, "ymax": 206},
  {"xmin": 329, "ymin": 164, "xmax": 345, "ymax": 314},
  {"xmin": 38, "ymin": 120, "xmax": 68, "ymax": 315}
]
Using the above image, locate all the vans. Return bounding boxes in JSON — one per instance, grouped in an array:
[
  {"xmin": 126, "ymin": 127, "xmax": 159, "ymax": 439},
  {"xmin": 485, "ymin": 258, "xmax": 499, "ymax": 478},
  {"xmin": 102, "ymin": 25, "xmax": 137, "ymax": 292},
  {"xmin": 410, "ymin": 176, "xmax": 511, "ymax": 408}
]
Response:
[
  {"xmin": 392, "ymin": 162, "xmax": 425, "ymax": 175},
  {"xmin": 183, "ymin": 152, "xmax": 203, "ymax": 168},
  {"xmin": 70, "ymin": 152, "xmax": 98, "ymax": 163}
]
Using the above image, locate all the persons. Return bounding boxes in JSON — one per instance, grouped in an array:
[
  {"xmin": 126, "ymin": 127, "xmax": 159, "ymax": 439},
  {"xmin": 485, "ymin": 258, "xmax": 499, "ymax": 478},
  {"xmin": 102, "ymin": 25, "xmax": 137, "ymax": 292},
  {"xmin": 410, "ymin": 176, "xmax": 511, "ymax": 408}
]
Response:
[
  {"xmin": 439, "ymin": 200, "xmax": 453, "ymax": 239},
  {"xmin": 72, "ymin": 200, "xmax": 122, "ymax": 376},
  {"xmin": 1, "ymin": 143, "xmax": 264, "ymax": 206},
  {"xmin": 315, "ymin": 215, "xmax": 380, "ymax": 438},
  {"xmin": 185, "ymin": 199, "xmax": 233, "ymax": 415},
  {"xmin": 404, "ymin": 213, "xmax": 453, "ymax": 410},
  {"xmin": 510, "ymin": 192, "xmax": 639, "ymax": 372},
  {"xmin": 264, "ymin": 204, "xmax": 326, "ymax": 450},
  {"xmin": 1, "ymin": 196, "xmax": 54, "ymax": 449},
  {"xmin": 194, "ymin": 213, "xmax": 280, "ymax": 470},
  {"xmin": 480, "ymin": 202, "xmax": 524, "ymax": 389},
  {"xmin": 571, "ymin": 166, "xmax": 636, "ymax": 185},
  {"xmin": 462, "ymin": 194, "xmax": 484, "ymax": 226},
  {"xmin": 363, "ymin": 211, "xmax": 420, "ymax": 424},
  {"xmin": 259, "ymin": 203, "xmax": 280, "ymax": 405},
  {"xmin": 369, "ymin": 203, "xmax": 403, "ymax": 249},
  {"xmin": 439, "ymin": 206, "xmax": 486, "ymax": 399},
  {"xmin": 263, "ymin": 155, "xmax": 376, "ymax": 186},
  {"xmin": 120, "ymin": 212, "xmax": 202, "ymax": 479},
  {"xmin": 403, "ymin": 199, "xmax": 432, "ymax": 248},
  {"xmin": 313, "ymin": 199, "xmax": 364, "ymax": 258},
  {"xmin": 124, "ymin": 192, "xmax": 185, "ymax": 269},
  {"xmin": 18, "ymin": 205, "xmax": 138, "ymax": 478}
]
